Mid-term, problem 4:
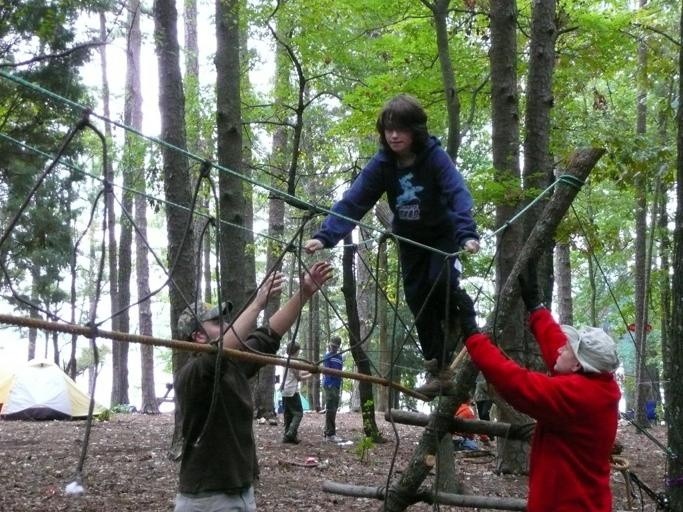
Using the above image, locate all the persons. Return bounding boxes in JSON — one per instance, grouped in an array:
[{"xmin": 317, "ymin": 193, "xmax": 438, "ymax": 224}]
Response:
[
  {"xmin": 470, "ymin": 371, "xmax": 494, "ymax": 442},
  {"xmin": 173, "ymin": 262, "xmax": 334, "ymax": 512},
  {"xmin": 451, "ymin": 258, "xmax": 622, "ymax": 512},
  {"xmin": 304, "ymin": 94, "xmax": 482, "ymax": 395},
  {"xmin": 452, "ymin": 392, "xmax": 478, "ymax": 450},
  {"xmin": 321, "ymin": 335, "xmax": 343, "ymax": 442},
  {"xmin": 280, "ymin": 341, "xmax": 313, "ymax": 445}
]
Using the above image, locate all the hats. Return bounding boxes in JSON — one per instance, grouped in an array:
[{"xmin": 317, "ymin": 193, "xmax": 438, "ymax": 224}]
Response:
[
  {"xmin": 560, "ymin": 324, "xmax": 619, "ymax": 375},
  {"xmin": 178, "ymin": 300, "xmax": 233, "ymax": 340},
  {"xmin": 330, "ymin": 336, "xmax": 341, "ymax": 346}
]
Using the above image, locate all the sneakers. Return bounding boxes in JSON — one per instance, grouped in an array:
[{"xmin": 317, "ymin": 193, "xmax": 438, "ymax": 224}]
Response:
[
  {"xmin": 282, "ymin": 432, "xmax": 298, "ymax": 444},
  {"xmin": 322, "ymin": 433, "xmax": 343, "ymax": 442}
]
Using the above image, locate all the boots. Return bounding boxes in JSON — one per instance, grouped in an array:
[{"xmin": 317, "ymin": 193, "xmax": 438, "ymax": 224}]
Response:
[
  {"xmin": 416, "ymin": 358, "xmax": 458, "ymax": 395},
  {"xmin": 440, "ymin": 317, "xmax": 462, "ymax": 351}
]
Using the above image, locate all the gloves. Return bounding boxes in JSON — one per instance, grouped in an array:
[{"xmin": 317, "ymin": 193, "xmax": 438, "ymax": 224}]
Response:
[
  {"xmin": 453, "ymin": 288, "xmax": 480, "ymax": 342},
  {"xmin": 518, "ymin": 257, "xmax": 541, "ymax": 310}
]
